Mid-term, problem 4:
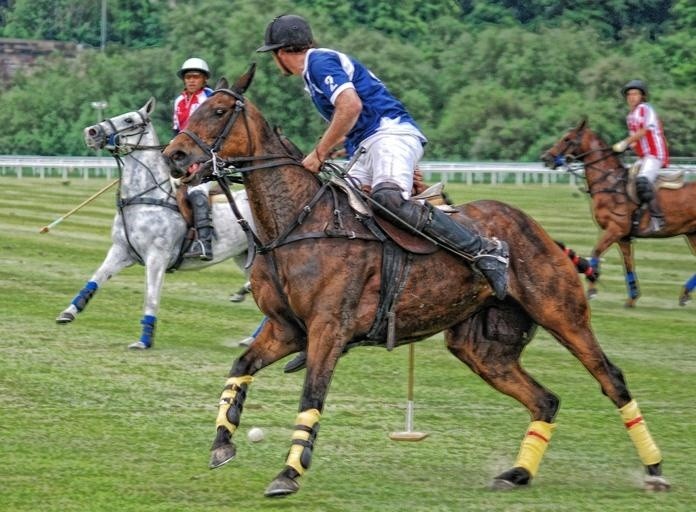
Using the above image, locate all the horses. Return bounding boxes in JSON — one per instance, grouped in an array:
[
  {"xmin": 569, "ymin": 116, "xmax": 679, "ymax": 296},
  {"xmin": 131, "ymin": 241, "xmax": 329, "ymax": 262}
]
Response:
[
  {"xmin": 539, "ymin": 113, "xmax": 696, "ymax": 309},
  {"xmin": 161, "ymin": 62, "xmax": 671, "ymax": 497},
  {"xmin": 56, "ymin": 96, "xmax": 269, "ymax": 351}
]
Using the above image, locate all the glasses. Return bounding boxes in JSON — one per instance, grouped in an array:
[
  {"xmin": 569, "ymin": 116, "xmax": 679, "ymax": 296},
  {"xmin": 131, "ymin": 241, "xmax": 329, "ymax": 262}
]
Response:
[{"xmin": 184, "ymin": 74, "xmax": 204, "ymax": 80}]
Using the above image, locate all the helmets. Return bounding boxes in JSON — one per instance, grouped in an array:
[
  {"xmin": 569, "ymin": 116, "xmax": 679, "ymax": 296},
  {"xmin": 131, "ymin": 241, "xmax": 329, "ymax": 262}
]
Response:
[
  {"xmin": 620, "ymin": 79, "xmax": 647, "ymax": 97},
  {"xmin": 177, "ymin": 57, "xmax": 211, "ymax": 80},
  {"xmin": 255, "ymin": 13, "xmax": 312, "ymax": 53}
]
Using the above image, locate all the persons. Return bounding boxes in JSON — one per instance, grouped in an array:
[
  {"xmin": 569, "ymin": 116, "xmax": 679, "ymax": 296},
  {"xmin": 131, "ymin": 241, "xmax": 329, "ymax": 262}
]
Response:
[
  {"xmin": 607, "ymin": 78, "xmax": 675, "ymax": 236},
  {"xmin": 161, "ymin": 54, "xmax": 228, "ymax": 264},
  {"xmin": 256, "ymin": 12, "xmax": 516, "ymax": 297}
]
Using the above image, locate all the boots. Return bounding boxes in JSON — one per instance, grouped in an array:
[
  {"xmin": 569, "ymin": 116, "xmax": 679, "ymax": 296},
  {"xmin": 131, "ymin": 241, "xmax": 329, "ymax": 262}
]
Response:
[
  {"xmin": 636, "ymin": 197, "xmax": 665, "ymax": 236},
  {"xmin": 181, "ymin": 188, "xmax": 213, "ymax": 261},
  {"xmin": 412, "ymin": 200, "xmax": 509, "ymax": 300}
]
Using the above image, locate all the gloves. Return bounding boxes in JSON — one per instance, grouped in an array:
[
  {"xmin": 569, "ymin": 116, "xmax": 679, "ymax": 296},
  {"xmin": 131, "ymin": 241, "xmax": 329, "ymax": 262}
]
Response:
[{"xmin": 611, "ymin": 140, "xmax": 627, "ymax": 152}]
